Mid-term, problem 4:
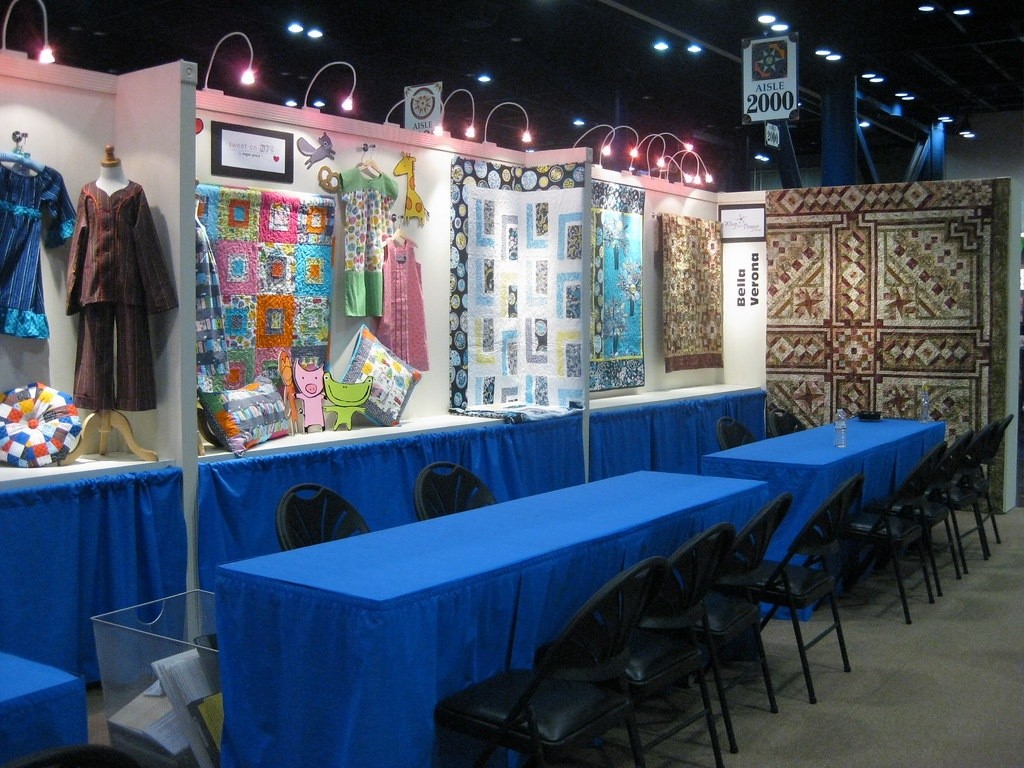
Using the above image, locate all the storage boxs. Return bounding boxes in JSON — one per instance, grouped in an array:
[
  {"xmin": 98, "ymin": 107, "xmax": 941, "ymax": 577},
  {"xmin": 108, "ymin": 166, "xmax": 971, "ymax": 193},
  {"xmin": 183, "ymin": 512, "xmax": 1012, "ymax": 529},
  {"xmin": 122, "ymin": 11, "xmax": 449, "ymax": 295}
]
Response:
[{"xmin": 90, "ymin": 589, "xmax": 223, "ymax": 768}]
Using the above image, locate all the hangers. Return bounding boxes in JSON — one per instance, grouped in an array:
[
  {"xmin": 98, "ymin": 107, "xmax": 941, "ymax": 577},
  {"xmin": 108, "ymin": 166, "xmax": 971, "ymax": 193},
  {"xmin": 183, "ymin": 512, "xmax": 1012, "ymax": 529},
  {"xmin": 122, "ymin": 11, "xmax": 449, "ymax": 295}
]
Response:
[
  {"xmin": 359, "ymin": 144, "xmax": 383, "ymax": 175},
  {"xmin": 0, "ymin": 134, "xmax": 45, "ymax": 174}
]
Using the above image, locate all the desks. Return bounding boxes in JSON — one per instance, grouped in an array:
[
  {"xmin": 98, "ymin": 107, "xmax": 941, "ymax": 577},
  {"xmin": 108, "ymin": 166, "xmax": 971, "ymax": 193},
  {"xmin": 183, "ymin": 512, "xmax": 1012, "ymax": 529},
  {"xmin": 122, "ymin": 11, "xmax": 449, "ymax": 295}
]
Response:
[
  {"xmin": 0, "ymin": 652, "xmax": 88, "ymax": 763},
  {"xmin": 701, "ymin": 418, "xmax": 946, "ymax": 622},
  {"xmin": 221, "ymin": 470, "xmax": 769, "ymax": 767}
]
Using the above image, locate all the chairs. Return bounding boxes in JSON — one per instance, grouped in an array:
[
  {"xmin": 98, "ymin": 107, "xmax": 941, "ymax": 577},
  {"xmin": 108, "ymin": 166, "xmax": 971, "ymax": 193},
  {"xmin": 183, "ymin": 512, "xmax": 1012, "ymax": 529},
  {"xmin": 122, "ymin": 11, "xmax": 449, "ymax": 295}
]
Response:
[
  {"xmin": 276, "ymin": 483, "xmax": 371, "ymax": 551},
  {"xmin": 414, "ymin": 407, "xmax": 1015, "ymax": 767}
]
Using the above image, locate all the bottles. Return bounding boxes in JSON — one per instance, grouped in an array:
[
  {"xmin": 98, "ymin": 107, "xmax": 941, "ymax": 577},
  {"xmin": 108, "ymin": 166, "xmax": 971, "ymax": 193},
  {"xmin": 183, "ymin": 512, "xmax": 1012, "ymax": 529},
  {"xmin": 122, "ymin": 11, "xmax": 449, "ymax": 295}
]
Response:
[
  {"xmin": 834, "ymin": 409, "xmax": 846, "ymax": 447},
  {"xmin": 919, "ymin": 381, "xmax": 931, "ymax": 424},
  {"xmin": 858, "ymin": 409, "xmax": 880, "ymax": 420}
]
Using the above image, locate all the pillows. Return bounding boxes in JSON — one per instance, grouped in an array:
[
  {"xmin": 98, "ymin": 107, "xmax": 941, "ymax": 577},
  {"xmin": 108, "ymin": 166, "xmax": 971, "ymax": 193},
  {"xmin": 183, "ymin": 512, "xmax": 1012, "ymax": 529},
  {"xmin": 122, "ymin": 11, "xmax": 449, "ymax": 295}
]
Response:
[
  {"xmin": 198, "ymin": 375, "xmax": 289, "ymax": 457},
  {"xmin": 340, "ymin": 324, "xmax": 423, "ymax": 428}
]
